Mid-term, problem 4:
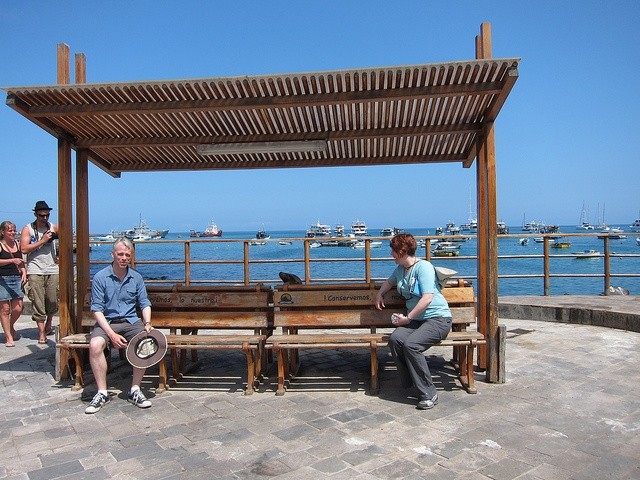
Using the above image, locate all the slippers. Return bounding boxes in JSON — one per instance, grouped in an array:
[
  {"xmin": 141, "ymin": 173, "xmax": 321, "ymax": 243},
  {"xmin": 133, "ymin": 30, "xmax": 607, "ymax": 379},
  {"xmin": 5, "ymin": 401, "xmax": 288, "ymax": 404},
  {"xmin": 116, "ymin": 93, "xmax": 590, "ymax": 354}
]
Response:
[
  {"xmin": 11, "ymin": 328, "xmax": 17, "ymax": 340},
  {"xmin": 46, "ymin": 322, "xmax": 52, "ymax": 335},
  {"xmin": 6, "ymin": 341, "xmax": 15, "ymax": 347},
  {"xmin": 38, "ymin": 336, "xmax": 48, "ymax": 344}
]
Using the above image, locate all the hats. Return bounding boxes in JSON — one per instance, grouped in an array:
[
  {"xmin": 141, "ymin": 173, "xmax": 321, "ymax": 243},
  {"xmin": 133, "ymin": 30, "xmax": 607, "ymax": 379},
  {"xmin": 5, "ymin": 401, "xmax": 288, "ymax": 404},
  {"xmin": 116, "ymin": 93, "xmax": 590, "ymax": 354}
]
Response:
[
  {"xmin": 433, "ymin": 266, "xmax": 460, "ymax": 290},
  {"xmin": 32, "ymin": 201, "xmax": 53, "ymax": 211},
  {"xmin": 126, "ymin": 329, "xmax": 168, "ymax": 369}
]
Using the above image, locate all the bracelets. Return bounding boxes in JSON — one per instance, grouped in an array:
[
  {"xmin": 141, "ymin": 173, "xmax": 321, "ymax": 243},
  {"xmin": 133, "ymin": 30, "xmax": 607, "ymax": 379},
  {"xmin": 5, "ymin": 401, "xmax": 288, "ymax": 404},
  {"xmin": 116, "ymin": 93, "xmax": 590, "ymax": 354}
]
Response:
[{"xmin": 407, "ymin": 315, "xmax": 413, "ymax": 322}]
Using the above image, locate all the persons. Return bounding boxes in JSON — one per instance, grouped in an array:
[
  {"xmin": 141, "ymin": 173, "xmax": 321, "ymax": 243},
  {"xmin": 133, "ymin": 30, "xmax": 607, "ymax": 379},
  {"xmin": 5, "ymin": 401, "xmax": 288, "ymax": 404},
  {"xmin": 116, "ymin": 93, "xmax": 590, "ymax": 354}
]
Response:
[
  {"xmin": 373, "ymin": 234, "xmax": 453, "ymax": 410},
  {"xmin": 19, "ymin": 201, "xmax": 59, "ymax": 343},
  {"xmin": 0, "ymin": 220, "xmax": 27, "ymax": 347},
  {"xmin": 84, "ymin": 237, "xmax": 153, "ymax": 413}
]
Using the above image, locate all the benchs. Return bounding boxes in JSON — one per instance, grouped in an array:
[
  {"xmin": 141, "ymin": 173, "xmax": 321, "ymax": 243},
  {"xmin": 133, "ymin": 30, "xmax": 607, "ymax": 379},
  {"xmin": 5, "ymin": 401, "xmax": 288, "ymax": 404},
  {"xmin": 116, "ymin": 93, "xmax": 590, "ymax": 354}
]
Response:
[
  {"xmin": 275, "ymin": 284, "xmax": 471, "ymax": 375},
  {"xmin": 55, "ymin": 289, "xmax": 267, "ymax": 395},
  {"xmin": 264, "ymin": 281, "xmax": 487, "ymax": 396},
  {"xmin": 81, "ymin": 285, "xmax": 272, "ymax": 375}
]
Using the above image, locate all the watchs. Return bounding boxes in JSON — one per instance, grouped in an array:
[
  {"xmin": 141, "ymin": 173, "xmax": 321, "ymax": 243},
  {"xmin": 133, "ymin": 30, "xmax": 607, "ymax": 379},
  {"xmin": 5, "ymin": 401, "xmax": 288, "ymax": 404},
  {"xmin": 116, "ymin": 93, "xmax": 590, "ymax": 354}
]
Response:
[{"xmin": 144, "ymin": 322, "xmax": 152, "ymax": 326}]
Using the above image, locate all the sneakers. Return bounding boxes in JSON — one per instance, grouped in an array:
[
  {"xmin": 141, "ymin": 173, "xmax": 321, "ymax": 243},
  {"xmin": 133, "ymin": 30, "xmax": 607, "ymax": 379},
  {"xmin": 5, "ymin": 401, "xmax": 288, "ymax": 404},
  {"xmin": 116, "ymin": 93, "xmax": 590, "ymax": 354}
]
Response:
[
  {"xmin": 127, "ymin": 389, "xmax": 152, "ymax": 408},
  {"xmin": 417, "ymin": 392, "xmax": 440, "ymax": 409},
  {"xmin": 85, "ymin": 392, "xmax": 111, "ymax": 414}
]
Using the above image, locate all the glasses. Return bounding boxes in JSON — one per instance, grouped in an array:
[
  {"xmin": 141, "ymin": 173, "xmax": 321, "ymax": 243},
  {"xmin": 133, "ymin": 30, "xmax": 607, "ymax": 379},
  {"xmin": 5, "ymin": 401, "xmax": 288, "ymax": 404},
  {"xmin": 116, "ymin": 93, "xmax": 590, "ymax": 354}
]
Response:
[
  {"xmin": 36, "ymin": 213, "xmax": 50, "ymax": 217},
  {"xmin": 400, "ymin": 283, "xmax": 413, "ymax": 300}
]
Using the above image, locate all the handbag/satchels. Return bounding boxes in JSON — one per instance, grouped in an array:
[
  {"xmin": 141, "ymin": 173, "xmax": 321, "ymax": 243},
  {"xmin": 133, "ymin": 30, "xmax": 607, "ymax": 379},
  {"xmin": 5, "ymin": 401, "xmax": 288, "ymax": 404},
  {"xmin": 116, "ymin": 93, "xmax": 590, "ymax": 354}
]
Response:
[{"xmin": 410, "ymin": 280, "xmax": 424, "ymax": 297}]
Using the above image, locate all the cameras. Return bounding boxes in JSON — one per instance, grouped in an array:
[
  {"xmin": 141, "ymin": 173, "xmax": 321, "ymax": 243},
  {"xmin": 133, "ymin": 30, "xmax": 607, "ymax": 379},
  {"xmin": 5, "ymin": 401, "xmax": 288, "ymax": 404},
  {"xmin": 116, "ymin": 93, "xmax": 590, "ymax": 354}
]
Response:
[{"xmin": 46, "ymin": 231, "xmax": 60, "ymax": 242}]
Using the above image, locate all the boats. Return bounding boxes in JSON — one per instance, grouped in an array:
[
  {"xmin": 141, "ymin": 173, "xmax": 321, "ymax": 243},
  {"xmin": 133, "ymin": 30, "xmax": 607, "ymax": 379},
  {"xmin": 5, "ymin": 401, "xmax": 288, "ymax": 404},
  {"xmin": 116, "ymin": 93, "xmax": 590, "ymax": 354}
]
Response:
[
  {"xmin": 438, "ymin": 226, "xmax": 471, "ymax": 241},
  {"xmin": 381, "ymin": 227, "xmax": 394, "ymax": 236},
  {"xmin": 597, "ymin": 227, "xmax": 627, "ymax": 239},
  {"xmin": 350, "ymin": 241, "xmax": 383, "ymax": 249},
  {"xmin": 434, "ymin": 242, "xmax": 460, "ymax": 250},
  {"xmin": 416, "ymin": 238, "xmax": 438, "ymax": 246},
  {"xmin": 571, "ymin": 249, "xmax": 600, "ymax": 259},
  {"xmin": 279, "ymin": 241, "xmax": 293, "ymax": 246},
  {"xmin": 533, "ymin": 237, "xmax": 555, "ymax": 242},
  {"xmin": 249, "ymin": 222, "xmax": 271, "ymax": 246},
  {"xmin": 550, "ymin": 236, "xmax": 571, "ymax": 247},
  {"xmin": 522, "ymin": 220, "xmax": 545, "ymax": 231},
  {"xmin": 518, "ymin": 238, "xmax": 530, "ymax": 247},
  {"xmin": 540, "ymin": 224, "xmax": 566, "ymax": 238},
  {"xmin": 334, "ymin": 221, "xmax": 345, "ymax": 237},
  {"xmin": 628, "ymin": 219, "xmax": 640, "ymax": 233},
  {"xmin": 430, "ymin": 245, "xmax": 460, "ymax": 257},
  {"xmin": 445, "ymin": 219, "xmax": 457, "ymax": 233},
  {"xmin": 435, "ymin": 225, "xmax": 443, "ymax": 235}
]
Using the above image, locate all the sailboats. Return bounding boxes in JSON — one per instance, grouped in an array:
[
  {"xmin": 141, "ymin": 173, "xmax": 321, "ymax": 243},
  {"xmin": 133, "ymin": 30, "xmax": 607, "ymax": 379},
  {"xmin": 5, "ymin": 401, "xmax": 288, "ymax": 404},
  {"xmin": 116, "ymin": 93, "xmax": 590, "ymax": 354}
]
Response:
[
  {"xmin": 576, "ymin": 198, "xmax": 594, "ymax": 230},
  {"xmin": 593, "ymin": 201, "xmax": 608, "ymax": 230},
  {"xmin": 460, "ymin": 182, "xmax": 510, "ymax": 235}
]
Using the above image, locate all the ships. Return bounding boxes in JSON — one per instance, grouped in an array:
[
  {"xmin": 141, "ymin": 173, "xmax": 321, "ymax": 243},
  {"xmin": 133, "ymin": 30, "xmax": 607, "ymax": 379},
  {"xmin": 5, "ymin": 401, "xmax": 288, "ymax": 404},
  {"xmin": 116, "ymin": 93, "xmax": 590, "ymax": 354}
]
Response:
[
  {"xmin": 350, "ymin": 218, "xmax": 368, "ymax": 235},
  {"xmin": 305, "ymin": 218, "xmax": 332, "ymax": 242},
  {"xmin": 91, "ymin": 224, "xmax": 161, "ymax": 245},
  {"xmin": 189, "ymin": 214, "xmax": 224, "ymax": 238},
  {"xmin": 119, "ymin": 211, "xmax": 170, "ymax": 240}
]
